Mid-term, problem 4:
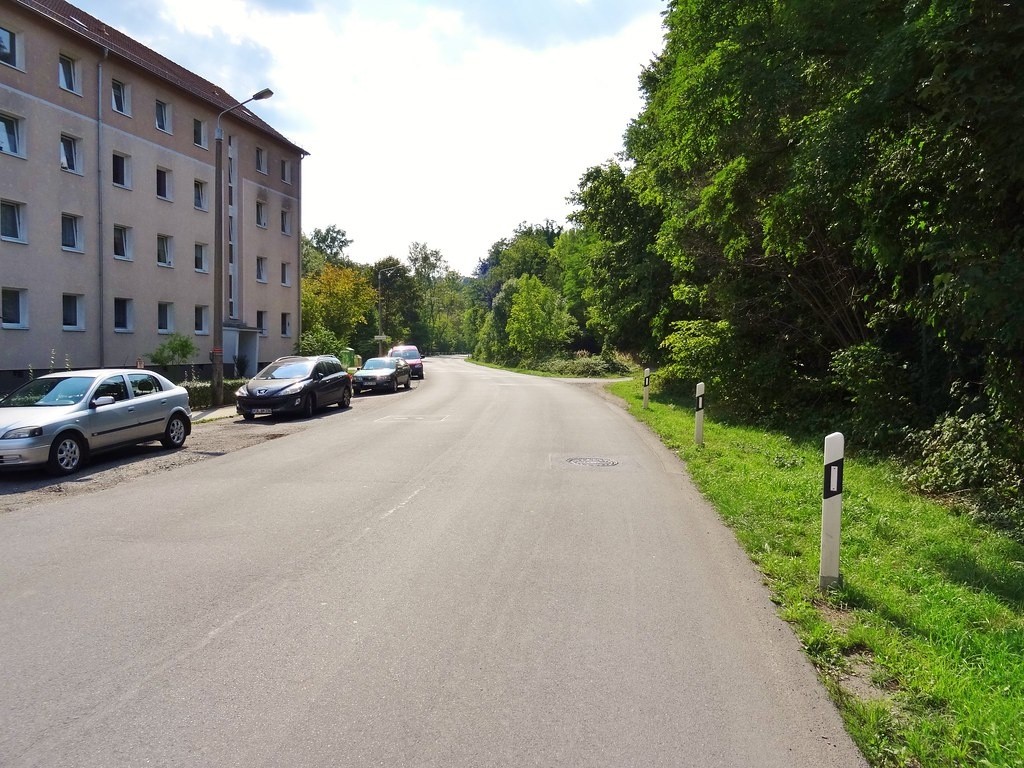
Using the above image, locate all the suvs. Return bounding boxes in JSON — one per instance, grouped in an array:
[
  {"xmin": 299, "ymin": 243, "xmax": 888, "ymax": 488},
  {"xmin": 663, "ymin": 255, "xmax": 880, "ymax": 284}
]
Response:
[
  {"xmin": 390, "ymin": 346, "xmax": 425, "ymax": 380},
  {"xmin": 236, "ymin": 354, "xmax": 353, "ymax": 421}
]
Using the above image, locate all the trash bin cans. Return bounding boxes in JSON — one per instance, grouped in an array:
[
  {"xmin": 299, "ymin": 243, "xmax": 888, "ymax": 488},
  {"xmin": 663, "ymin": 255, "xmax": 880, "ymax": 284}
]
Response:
[
  {"xmin": 341, "ymin": 347, "xmax": 354, "ymax": 368},
  {"xmin": 354, "ymin": 355, "xmax": 362, "ymax": 368}
]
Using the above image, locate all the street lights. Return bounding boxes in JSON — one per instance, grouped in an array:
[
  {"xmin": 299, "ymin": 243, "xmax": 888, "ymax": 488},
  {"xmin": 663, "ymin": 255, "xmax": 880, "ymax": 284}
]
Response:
[{"xmin": 210, "ymin": 88, "xmax": 275, "ymax": 408}]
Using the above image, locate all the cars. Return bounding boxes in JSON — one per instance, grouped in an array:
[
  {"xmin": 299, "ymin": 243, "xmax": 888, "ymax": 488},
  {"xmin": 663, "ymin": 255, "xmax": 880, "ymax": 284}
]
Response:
[
  {"xmin": 351, "ymin": 356, "xmax": 412, "ymax": 394},
  {"xmin": 0, "ymin": 369, "xmax": 194, "ymax": 479}
]
